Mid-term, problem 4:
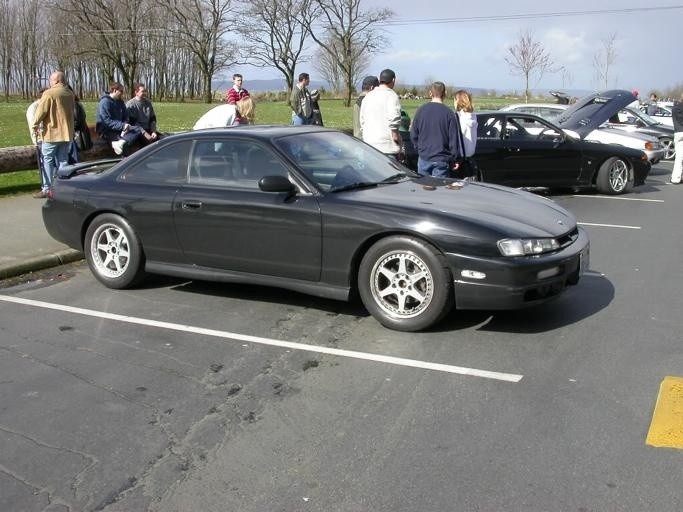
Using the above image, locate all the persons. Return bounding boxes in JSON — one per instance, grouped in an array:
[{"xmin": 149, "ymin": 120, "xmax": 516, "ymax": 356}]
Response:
[
  {"xmin": 226, "ymin": 73, "xmax": 249, "ymax": 127},
  {"xmin": 650, "ymin": 93, "xmax": 656, "ymax": 102},
  {"xmin": 65, "ymin": 82, "xmax": 92, "ymax": 166},
  {"xmin": 309, "ymin": 89, "xmax": 324, "ymax": 126},
  {"xmin": 288, "ymin": 72, "xmax": 313, "ymax": 125},
  {"xmin": 31, "ymin": 71, "xmax": 76, "ymax": 198},
  {"xmin": 409, "ymin": 81, "xmax": 460, "ymax": 178},
  {"xmin": 25, "ymin": 86, "xmax": 60, "ymax": 179},
  {"xmin": 411, "ymin": 175, "xmax": 466, "ymax": 192},
  {"xmin": 359, "ymin": 68, "xmax": 403, "ymax": 166},
  {"xmin": 92, "ymin": 81, "xmax": 143, "ymax": 157},
  {"xmin": 670, "ymin": 95, "xmax": 682, "ymax": 184},
  {"xmin": 191, "ymin": 96, "xmax": 255, "ymax": 152},
  {"xmin": 626, "ymin": 90, "xmax": 641, "ymax": 123},
  {"xmin": 124, "ymin": 82, "xmax": 161, "ymax": 148},
  {"xmin": 452, "ymin": 89, "xmax": 478, "ymax": 180},
  {"xmin": 351, "ymin": 75, "xmax": 378, "ymax": 140}
]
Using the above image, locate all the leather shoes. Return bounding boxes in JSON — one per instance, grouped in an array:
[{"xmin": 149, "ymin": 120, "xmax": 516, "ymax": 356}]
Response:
[{"xmin": 33, "ymin": 192, "xmax": 50, "ymax": 199}]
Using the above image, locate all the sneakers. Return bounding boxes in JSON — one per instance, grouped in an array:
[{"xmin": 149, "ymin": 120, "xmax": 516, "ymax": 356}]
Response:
[{"xmin": 112, "ymin": 139, "xmax": 123, "ymax": 155}]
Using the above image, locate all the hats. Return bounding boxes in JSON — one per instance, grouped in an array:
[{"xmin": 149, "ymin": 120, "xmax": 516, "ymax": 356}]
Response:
[
  {"xmin": 362, "ymin": 76, "xmax": 379, "ymax": 86},
  {"xmin": 633, "ymin": 91, "xmax": 638, "ymax": 96},
  {"xmin": 380, "ymin": 69, "xmax": 396, "ymax": 83},
  {"xmin": 310, "ymin": 90, "xmax": 320, "ymax": 97}
]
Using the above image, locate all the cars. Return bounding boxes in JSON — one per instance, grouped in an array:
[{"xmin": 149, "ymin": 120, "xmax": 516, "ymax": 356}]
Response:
[
  {"xmin": 391, "ymin": 90, "xmax": 676, "ymax": 195},
  {"xmin": 37, "ymin": 124, "xmax": 592, "ymax": 333}
]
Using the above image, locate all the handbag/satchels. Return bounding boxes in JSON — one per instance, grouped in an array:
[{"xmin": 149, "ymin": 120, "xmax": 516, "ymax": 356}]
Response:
[{"xmin": 449, "ymin": 155, "xmax": 476, "ymax": 178}]
